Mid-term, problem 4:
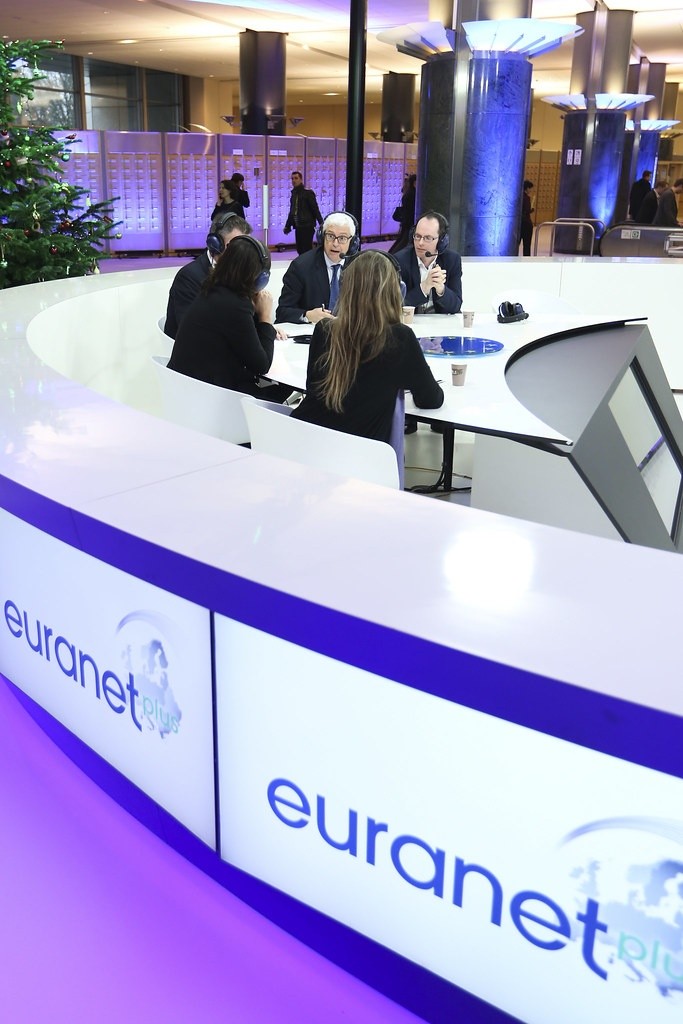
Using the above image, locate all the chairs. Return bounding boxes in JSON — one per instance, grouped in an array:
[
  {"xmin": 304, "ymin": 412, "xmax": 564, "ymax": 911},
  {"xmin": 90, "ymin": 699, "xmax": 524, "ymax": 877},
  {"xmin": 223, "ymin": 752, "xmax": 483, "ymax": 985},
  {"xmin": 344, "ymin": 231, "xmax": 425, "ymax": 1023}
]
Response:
[
  {"xmin": 154, "ymin": 314, "xmax": 175, "ymax": 359},
  {"xmin": 147, "ymin": 356, "xmax": 296, "ymax": 444},
  {"xmin": 240, "ymin": 396, "xmax": 400, "ymax": 489}
]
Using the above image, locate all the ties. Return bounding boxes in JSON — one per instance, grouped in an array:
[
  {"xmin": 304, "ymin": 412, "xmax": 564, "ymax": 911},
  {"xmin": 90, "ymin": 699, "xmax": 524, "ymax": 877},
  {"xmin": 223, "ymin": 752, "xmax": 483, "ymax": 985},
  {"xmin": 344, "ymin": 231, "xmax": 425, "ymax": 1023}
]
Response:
[{"xmin": 328, "ymin": 264, "xmax": 340, "ymax": 313}]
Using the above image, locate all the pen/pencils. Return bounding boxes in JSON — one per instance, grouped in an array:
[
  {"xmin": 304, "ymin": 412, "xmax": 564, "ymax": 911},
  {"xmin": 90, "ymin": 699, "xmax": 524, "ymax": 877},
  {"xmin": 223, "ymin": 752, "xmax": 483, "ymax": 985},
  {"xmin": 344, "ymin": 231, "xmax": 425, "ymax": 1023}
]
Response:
[{"xmin": 322, "ymin": 303, "xmax": 325, "ymax": 312}]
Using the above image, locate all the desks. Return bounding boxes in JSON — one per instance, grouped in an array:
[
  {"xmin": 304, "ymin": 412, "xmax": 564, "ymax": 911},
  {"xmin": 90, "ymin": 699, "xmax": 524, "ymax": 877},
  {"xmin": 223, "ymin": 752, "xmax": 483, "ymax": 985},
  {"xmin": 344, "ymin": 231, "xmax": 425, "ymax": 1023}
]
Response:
[{"xmin": 261, "ymin": 314, "xmax": 683, "ymax": 554}]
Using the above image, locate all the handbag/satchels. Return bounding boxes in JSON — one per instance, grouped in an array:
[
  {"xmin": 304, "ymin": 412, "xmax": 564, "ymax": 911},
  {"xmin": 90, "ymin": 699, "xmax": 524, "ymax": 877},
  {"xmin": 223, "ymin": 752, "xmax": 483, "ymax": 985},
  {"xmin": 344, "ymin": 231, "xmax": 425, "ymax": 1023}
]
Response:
[{"xmin": 392, "ymin": 207, "xmax": 404, "ymax": 221}]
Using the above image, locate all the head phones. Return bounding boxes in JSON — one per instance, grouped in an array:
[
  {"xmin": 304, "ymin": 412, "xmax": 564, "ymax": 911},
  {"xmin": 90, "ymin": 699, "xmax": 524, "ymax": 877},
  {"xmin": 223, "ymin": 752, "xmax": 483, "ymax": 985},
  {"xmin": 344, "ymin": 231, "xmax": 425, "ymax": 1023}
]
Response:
[
  {"xmin": 226, "ymin": 234, "xmax": 270, "ymax": 291},
  {"xmin": 342, "ymin": 249, "xmax": 406, "ymax": 304},
  {"xmin": 316, "ymin": 211, "xmax": 360, "ymax": 259},
  {"xmin": 497, "ymin": 301, "xmax": 529, "ymax": 323},
  {"xmin": 205, "ymin": 212, "xmax": 237, "ymax": 257},
  {"xmin": 408, "ymin": 211, "xmax": 450, "ymax": 257}
]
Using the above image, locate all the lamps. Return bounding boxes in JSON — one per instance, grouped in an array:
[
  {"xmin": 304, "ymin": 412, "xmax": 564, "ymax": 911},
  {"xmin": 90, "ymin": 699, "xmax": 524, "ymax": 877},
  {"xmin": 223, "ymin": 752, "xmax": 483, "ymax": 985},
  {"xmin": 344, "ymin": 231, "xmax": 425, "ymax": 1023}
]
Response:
[
  {"xmin": 414, "ymin": 133, "xmax": 418, "ymax": 140},
  {"xmin": 527, "ymin": 139, "xmax": 541, "ymax": 149},
  {"xmin": 286, "ymin": 117, "xmax": 305, "ymax": 130},
  {"xmin": 402, "ymin": 131, "xmax": 414, "ymax": 141},
  {"xmin": 220, "ymin": 116, "xmax": 242, "ymax": 129},
  {"xmin": 268, "ymin": 114, "xmax": 285, "ymax": 129},
  {"xmin": 367, "ymin": 132, "xmax": 384, "ymax": 142}
]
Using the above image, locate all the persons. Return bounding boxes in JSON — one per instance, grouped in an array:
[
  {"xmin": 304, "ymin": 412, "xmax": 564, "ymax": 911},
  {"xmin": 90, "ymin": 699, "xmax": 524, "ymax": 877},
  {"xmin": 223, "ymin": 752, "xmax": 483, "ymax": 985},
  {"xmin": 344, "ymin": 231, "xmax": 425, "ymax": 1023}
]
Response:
[
  {"xmin": 230, "ymin": 173, "xmax": 249, "ymax": 208},
  {"xmin": 165, "ymin": 234, "xmax": 276, "ymax": 400},
  {"xmin": 283, "ymin": 172, "xmax": 323, "ymax": 256},
  {"xmin": 294, "ymin": 249, "xmax": 443, "ymax": 493},
  {"xmin": 634, "ymin": 181, "xmax": 668, "ymax": 224},
  {"xmin": 519, "ymin": 180, "xmax": 535, "ymax": 256},
  {"xmin": 651, "ymin": 178, "xmax": 683, "ymax": 228},
  {"xmin": 210, "ymin": 180, "xmax": 245, "ymax": 220},
  {"xmin": 272, "ymin": 211, "xmax": 360, "ymax": 323},
  {"xmin": 387, "ymin": 174, "xmax": 416, "ymax": 254},
  {"xmin": 628, "ymin": 171, "xmax": 652, "ymax": 222},
  {"xmin": 391, "ymin": 211, "xmax": 462, "ymax": 315},
  {"xmin": 164, "ymin": 212, "xmax": 288, "ymax": 341}
]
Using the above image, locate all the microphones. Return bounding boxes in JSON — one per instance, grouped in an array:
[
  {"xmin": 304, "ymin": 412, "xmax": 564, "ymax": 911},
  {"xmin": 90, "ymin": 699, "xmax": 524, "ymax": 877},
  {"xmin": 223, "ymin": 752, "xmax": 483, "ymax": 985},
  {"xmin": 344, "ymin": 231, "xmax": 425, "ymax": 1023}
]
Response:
[
  {"xmin": 425, "ymin": 245, "xmax": 448, "ymax": 257},
  {"xmin": 339, "ymin": 246, "xmax": 359, "ymax": 259}
]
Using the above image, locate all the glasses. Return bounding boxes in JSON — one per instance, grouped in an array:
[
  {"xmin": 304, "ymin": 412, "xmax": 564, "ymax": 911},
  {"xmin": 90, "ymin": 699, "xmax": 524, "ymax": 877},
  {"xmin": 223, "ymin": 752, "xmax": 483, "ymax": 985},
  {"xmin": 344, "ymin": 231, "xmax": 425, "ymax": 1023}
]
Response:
[
  {"xmin": 325, "ymin": 232, "xmax": 352, "ymax": 244},
  {"xmin": 412, "ymin": 233, "xmax": 439, "ymax": 242}
]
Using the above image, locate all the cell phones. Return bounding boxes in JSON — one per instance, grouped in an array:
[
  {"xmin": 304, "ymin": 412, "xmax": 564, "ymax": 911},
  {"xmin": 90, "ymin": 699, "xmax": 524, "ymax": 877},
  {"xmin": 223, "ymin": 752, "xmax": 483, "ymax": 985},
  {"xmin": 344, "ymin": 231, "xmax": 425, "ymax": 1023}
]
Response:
[{"xmin": 218, "ymin": 193, "xmax": 223, "ymax": 199}]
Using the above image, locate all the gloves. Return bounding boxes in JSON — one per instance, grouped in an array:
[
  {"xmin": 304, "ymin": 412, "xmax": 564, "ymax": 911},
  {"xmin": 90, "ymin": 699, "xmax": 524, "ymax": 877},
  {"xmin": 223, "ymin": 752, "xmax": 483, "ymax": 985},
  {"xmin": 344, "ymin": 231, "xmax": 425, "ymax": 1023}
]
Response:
[{"xmin": 283, "ymin": 226, "xmax": 291, "ymax": 234}]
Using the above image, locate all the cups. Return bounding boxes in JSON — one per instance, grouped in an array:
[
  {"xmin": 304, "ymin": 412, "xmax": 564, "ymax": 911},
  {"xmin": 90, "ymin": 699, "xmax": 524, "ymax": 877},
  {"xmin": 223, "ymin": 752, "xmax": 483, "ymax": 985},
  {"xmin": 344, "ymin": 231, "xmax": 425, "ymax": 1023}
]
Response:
[
  {"xmin": 451, "ymin": 365, "xmax": 467, "ymax": 386},
  {"xmin": 402, "ymin": 305, "xmax": 416, "ymax": 325},
  {"xmin": 463, "ymin": 311, "xmax": 474, "ymax": 328}
]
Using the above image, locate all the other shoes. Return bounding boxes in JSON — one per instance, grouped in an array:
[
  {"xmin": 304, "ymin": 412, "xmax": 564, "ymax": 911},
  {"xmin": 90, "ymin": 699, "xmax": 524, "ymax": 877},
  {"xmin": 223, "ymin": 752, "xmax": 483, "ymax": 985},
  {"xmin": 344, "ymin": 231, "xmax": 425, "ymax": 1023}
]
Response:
[{"xmin": 404, "ymin": 425, "xmax": 417, "ymax": 434}]
